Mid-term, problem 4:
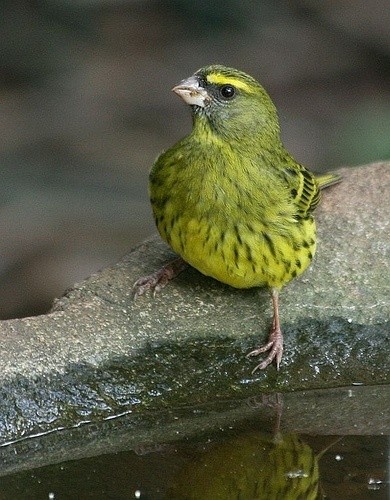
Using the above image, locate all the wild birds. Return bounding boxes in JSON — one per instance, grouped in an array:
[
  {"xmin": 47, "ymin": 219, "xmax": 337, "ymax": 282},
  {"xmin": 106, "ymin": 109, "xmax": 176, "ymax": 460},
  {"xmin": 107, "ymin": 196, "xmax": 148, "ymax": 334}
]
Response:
[{"xmin": 147, "ymin": 65, "xmax": 344, "ymax": 375}]
